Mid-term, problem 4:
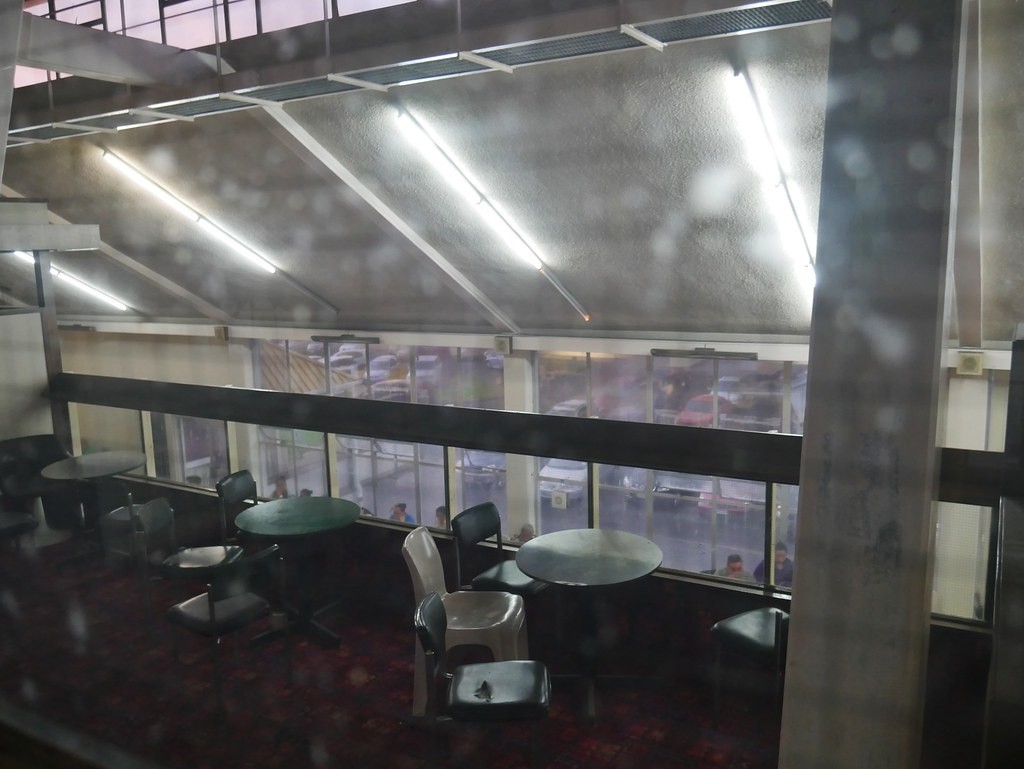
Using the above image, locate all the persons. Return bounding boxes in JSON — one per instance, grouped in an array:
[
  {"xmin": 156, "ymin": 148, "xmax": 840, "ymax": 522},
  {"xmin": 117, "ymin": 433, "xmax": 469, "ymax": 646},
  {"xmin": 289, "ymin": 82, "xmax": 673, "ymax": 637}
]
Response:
[
  {"xmin": 186, "ymin": 475, "xmax": 201, "ymax": 484},
  {"xmin": 300, "ymin": 489, "xmax": 313, "ymax": 497},
  {"xmin": 510, "ymin": 524, "xmax": 537, "ymax": 543},
  {"xmin": 753, "ymin": 543, "xmax": 794, "ymax": 588},
  {"xmin": 272, "ymin": 475, "xmax": 288, "ymax": 499},
  {"xmin": 436, "ymin": 505, "xmax": 446, "ymax": 529},
  {"xmin": 715, "ymin": 554, "xmax": 754, "ymax": 581},
  {"xmin": 390, "ymin": 503, "xmax": 415, "ymax": 523},
  {"xmin": 654, "ymin": 379, "xmax": 682, "ymax": 412}
]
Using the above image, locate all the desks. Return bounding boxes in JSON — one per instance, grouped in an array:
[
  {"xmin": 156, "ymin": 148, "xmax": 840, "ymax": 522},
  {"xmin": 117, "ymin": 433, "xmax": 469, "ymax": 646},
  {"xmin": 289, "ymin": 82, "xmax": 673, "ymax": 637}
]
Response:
[
  {"xmin": 516, "ymin": 527, "xmax": 664, "ymax": 727},
  {"xmin": 44, "ymin": 451, "xmax": 147, "ymax": 517},
  {"xmin": 235, "ymin": 495, "xmax": 360, "ymax": 647}
]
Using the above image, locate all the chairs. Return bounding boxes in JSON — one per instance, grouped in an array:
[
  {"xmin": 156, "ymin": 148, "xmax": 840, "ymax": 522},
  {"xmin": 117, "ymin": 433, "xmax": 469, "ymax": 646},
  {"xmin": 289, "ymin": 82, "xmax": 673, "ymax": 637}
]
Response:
[
  {"xmin": 709, "ymin": 605, "xmax": 788, "ymax": 727},
  {"xmin": 400, "ymin": 524, "xmax": 528, "ymax": 719},
  {"xmin": 452, "ymin": 500, "xmax": 562, "ymax": 659},
  {"xmin": 409, "ymin": 593, "xmax": 552, "ymax": 769},
  {"xmin": 0, "ymin": 437, "xmax": 261, "ymax": 666}
]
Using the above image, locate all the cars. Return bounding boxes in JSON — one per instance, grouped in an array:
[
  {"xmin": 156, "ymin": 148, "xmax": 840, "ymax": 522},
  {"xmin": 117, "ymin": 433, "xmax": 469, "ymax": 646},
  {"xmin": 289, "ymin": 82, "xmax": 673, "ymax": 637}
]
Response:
[
  {"xmin": 306, "ymin": 342, "xmax": 444, "ymax": 387},
  {"xmin": 623, "ymin": 366, "xmax": 809, "ymax": 517},
  {"xmin": 484, "ymin": 349, "xmax": 504, "ymax": 370},
  {"xmin": 454, "ymin": 450, "xmax": 506, "ymax": 486},
  {"xmin": 537, "ymin": 457, "xmax": 588, "ymax": 509},
  {"xmin": 547, "ymin": 396, "xmax": 587, "ymax": 417}
]
